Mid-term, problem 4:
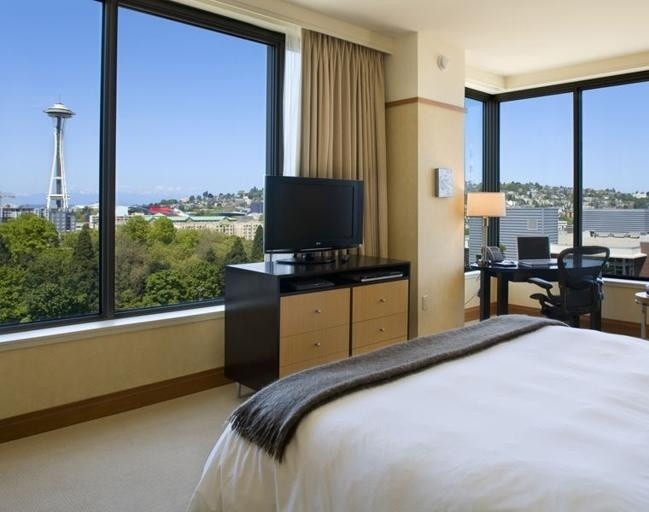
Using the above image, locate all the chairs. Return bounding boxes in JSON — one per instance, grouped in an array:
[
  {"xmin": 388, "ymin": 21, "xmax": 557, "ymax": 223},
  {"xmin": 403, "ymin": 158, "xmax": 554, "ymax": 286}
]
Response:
[{"xmin": 529, "ymin": 246, "xmax": 610, "ymax": 333}]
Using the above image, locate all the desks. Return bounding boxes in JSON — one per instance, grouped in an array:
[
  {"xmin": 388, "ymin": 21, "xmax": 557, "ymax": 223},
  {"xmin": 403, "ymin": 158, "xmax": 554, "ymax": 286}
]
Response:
[
  {"xmin": 634, "ymin": 292, "xmax": 649, "ymax": 340},
  {"xmin": 470, "ymin": 258, "xmax": 608, "ymax": 331}
]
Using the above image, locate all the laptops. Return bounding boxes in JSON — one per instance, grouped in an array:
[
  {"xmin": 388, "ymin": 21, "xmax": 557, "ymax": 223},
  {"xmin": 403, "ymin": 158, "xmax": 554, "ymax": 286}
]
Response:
[{"xmin": 517, "ymin": 236, "xmax": 567, "ymax": 267}]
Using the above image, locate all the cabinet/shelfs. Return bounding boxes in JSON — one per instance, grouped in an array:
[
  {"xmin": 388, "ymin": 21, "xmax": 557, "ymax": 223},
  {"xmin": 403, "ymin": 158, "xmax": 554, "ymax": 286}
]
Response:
[{"xmin": 225, "ymin": 254, "xmax": 410, "ymax": 398}]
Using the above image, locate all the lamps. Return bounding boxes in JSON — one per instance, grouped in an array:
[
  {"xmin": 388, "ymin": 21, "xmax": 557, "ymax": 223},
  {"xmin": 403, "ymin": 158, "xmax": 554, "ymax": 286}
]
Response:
[{"xmin": 467, "ymin": 192, "xmax": 506, "ymax": 264}]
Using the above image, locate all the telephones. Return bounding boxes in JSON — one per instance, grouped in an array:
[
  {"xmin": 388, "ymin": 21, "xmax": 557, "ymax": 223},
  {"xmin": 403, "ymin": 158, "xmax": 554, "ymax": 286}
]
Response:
[{"xmin": 481, "ymin": 245, "xmax": 503, "ymax": 262}]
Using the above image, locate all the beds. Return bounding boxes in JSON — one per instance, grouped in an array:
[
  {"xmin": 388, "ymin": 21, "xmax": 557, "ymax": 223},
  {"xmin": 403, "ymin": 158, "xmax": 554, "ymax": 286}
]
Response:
[{"xmin": 188, "ymin": 313, "xmax": 649, "ymax": 512}]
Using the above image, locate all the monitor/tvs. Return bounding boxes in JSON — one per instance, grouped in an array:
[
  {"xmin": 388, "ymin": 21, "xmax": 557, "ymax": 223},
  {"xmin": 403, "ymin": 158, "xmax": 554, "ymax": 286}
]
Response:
[{"xmin": 263, "ymin": 176, "xmax": 364, "ymax": 264}]
